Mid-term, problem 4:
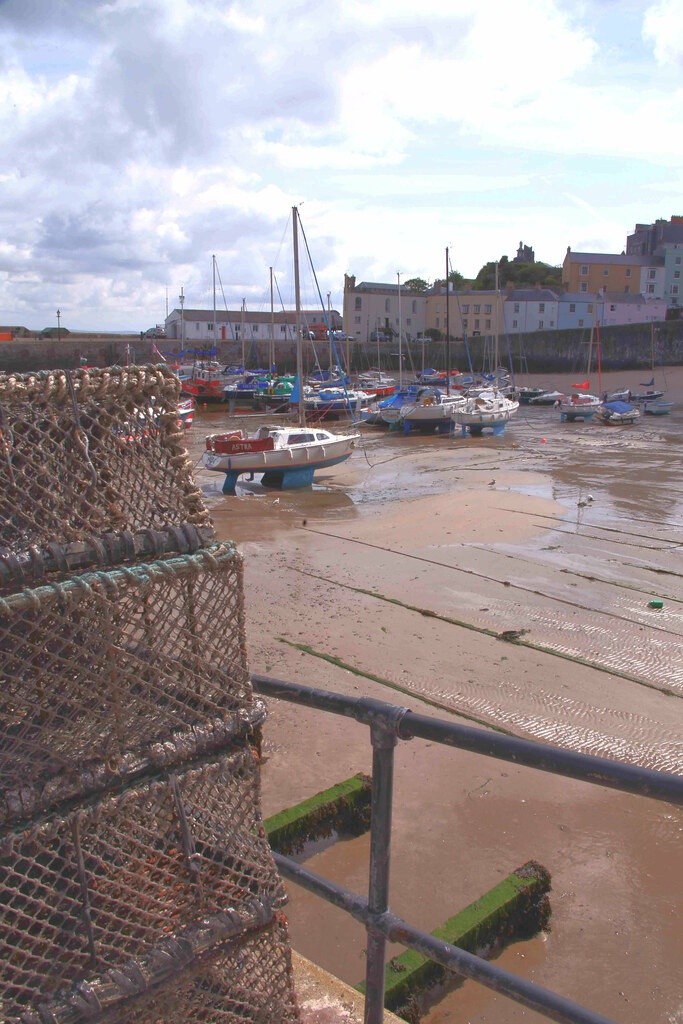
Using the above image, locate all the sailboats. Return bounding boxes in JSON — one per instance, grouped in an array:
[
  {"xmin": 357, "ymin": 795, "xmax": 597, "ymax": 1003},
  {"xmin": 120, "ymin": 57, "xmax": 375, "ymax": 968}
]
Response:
[
  {"xmin": 82, "ymin": 242, "xmax": 673, "ymax": 451},
  {"xmin": 200, "ymin": 206, "xmax": 363, "ymax": 495}
]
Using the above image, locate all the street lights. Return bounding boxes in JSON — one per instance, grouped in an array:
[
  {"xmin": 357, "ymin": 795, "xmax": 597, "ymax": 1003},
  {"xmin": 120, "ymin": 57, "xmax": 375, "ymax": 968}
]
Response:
[{"xmin": 56, "ymin": 309, "xmax": 61, "ymax": 343}]
